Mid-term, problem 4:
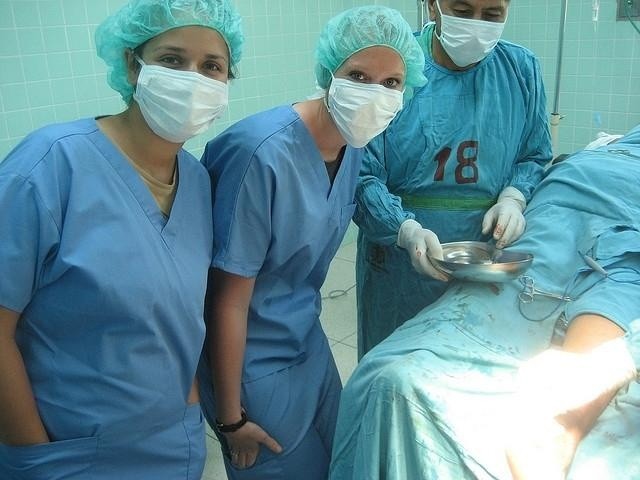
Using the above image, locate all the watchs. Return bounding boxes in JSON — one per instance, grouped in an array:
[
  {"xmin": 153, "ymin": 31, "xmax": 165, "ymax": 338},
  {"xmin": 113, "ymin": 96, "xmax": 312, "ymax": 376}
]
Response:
[{"xmin": 214, "ymin": 406, "xmax": 247, "ymax": 434}]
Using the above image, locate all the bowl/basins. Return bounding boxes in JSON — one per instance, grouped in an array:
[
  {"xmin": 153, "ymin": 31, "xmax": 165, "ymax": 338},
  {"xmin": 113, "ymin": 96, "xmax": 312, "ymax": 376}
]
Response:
[{"xmin": 426, "ymin": 240, "xmax": 535, "ymax": 285}]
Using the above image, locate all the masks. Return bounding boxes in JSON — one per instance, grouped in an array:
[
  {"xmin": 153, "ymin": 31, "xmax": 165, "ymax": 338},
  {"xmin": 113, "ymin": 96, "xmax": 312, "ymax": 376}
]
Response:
[
  {"xmin": 433, "ymin": 0, "xmax": 509, "ymax": 68},
  {"xmin": 324, "ymin": 74, "xmax": 407, "ymax": 150},
  {"xmin": 131, "ymin": 55, "xmax": 229, "ymax": 143}
]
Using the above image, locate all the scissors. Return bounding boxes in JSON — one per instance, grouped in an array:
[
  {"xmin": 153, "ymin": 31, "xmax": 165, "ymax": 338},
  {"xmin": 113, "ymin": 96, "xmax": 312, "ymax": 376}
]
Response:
[{"xmin": 518, "ymin": 275, "xmax": 577, "ymax": 304}]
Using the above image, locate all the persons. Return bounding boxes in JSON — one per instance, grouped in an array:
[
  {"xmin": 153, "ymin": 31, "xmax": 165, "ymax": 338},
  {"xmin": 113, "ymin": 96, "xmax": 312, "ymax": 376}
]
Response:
[
  {"xmin": 0, "ymin": 0, "xmax": 247, "ymax": 480},
  {"xmin": 351, "ymin": 0, "xmax": 555, "ymax": 365},
  {"xmin": 194, "ymin": 4, "xmax": 431, "ymax": 480},
  {"xmin": 366, "ymin": 120, "xmax": 640, "ymax": 480},
  {"xmin": 487, "ymin": 316, "xmax": 640, "ymax": 427}
]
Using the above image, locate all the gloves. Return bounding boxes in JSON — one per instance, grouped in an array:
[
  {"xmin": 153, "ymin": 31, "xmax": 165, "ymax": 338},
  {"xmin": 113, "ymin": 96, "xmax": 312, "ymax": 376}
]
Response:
[
  {"xmin": 399, "ymin": 218, "xmax": 451, "ymax": 283},
  {"xmin": 482, "ymin": 186, "xmax": 526, "ymax": 249}
]
